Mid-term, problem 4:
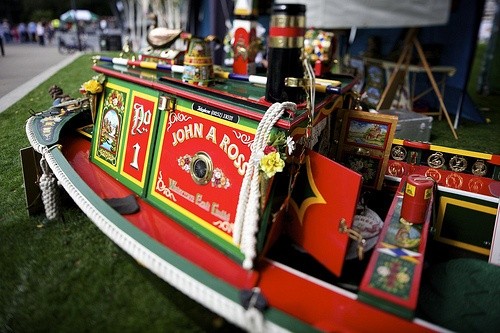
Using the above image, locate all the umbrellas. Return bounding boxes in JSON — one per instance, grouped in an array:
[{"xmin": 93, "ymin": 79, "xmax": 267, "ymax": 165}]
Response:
[{"xmin": 61, "ymin": 8, "xmax": 98, "ymax": 51}]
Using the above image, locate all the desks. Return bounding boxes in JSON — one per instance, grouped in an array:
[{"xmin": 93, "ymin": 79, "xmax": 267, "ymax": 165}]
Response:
[{"xmin": 362, "ymin": 56, "xmax": 456, "ymax": 121}]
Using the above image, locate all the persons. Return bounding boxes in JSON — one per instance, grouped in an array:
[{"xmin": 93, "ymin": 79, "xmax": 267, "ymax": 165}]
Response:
[{"xmin": 4, "ymin": 18, "xmax": 56, "ymax": 48}]
